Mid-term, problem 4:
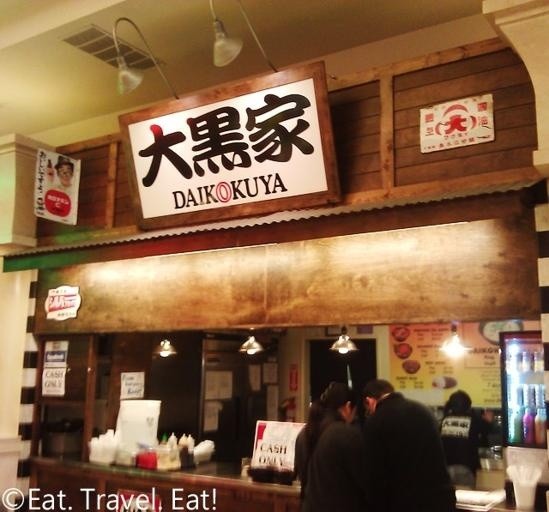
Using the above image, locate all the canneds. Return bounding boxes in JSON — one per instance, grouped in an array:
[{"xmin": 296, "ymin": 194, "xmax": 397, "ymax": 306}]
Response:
[
  {"xmin": 512, "ymin": 384, "xmax": 545, "ymax": 409},
  {"xmin": 511, "ymin": 351, "xmax": 544, "ymax": 373}
]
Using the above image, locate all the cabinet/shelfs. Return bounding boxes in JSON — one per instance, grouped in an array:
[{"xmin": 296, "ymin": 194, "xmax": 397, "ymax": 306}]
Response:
[{"xmin": 499, "ymin": 330, "xmax": 549, "ymax": 449}]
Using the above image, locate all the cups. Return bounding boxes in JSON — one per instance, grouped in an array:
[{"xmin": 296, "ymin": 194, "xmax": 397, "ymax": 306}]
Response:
[{"xmin": 510, "ymin": 481, "xmax": 539, "ymax": 511}]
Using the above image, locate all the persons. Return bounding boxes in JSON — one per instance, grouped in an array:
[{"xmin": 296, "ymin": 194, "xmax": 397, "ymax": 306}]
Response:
[
  {"xmin": 47, "ymin": 155, "xmax": 74, "ymax": 195},
  {"xmin": 439, "ymin": 389, "xmax": 503, "ymax": 489},
  {"xmin": 293, "ymin": 381, "xmax": 368, "ymax": 512},
  {"xmin": 361, "ymin": 379, "xmax": 458, "ymax": 512}
]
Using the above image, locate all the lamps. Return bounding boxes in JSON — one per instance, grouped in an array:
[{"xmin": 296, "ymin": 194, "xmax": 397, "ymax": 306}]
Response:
[
  {"xmin": 208, "ymin": 0, "xmax": 278, "ymax": 74},
  {"xmin": 439, "ymin": 322, "xmax": 469, "ymax": 353},
  {"xmin": 151, "ymin": 332, "xmax": 178, "ymax": 358},
  {"xmin": 329, "ymin": 325, "xmax": 358, "ymax": 355},
  {"xmin": 112, "ymin": 17, "xmax": 180, "ymax": 101},
  {"xmin": 238, "ymin": 328, "xmax": 265, "ymax": 356}
]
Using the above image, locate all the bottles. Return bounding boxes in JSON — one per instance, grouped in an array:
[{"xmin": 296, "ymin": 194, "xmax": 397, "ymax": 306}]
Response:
[
  {"xmin": 506, "ymin": 349, "xmax": 548, "ymax": 446},
  {"xmin": 156, "ymin": 432, "xmax": 196, "ymax": 471}
]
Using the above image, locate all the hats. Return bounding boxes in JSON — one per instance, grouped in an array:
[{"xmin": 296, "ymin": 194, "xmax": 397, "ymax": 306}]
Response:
[{"xmin": 55, "ymin": 155, "xmax": 73, "ymax": 168}]
